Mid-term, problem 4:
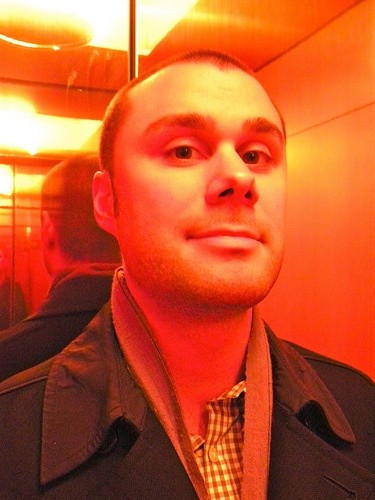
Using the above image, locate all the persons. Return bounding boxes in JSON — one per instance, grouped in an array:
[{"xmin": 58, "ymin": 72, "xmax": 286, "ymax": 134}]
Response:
[
  {"xmin": 2, "ymin": 153, "xmax": 123, "ymax": 385},
  {"xmin": 0, "ymin": 50, "xmax": 373, "ymax": 500}
]
